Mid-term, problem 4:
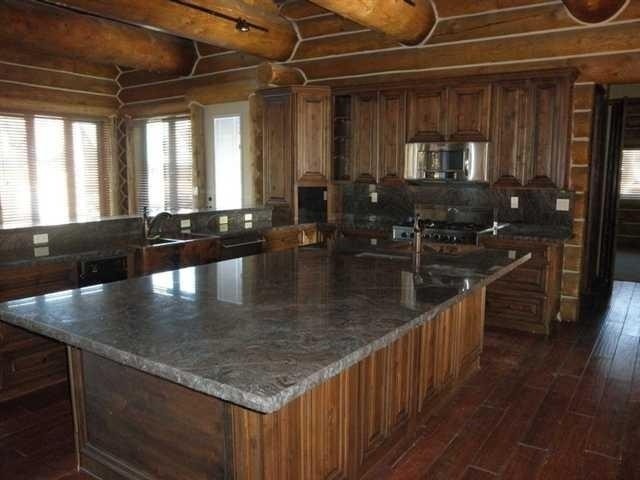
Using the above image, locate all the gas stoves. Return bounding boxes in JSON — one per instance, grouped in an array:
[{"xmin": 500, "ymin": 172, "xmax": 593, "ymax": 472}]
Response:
[{"xmin": 392, "ymin": 218, "xmax": 510, "ymax": 248}]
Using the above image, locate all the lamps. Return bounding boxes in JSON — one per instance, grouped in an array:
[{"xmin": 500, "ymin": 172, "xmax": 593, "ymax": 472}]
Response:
[{"xmin": 237, "ymin": 18, "xmax": 249, "ymax": 31}]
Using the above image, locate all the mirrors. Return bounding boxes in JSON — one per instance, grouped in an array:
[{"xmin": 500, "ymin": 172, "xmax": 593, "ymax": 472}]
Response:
[{"xmin": 0, "ymin": 0, "xmax": 263, "ymax": 230}]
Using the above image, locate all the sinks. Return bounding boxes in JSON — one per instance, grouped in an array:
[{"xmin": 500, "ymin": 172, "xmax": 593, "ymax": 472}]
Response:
[
  {"xmin": 166, "ymin": 231, "xmax": 209, "ymax": 241},
  {"xmin": 127, "ymin": 238, "xmax": 176, "ymax": 246},
  {"xmin": 376, "ymin": 238, "xmax": 486, "ymax": 263}
]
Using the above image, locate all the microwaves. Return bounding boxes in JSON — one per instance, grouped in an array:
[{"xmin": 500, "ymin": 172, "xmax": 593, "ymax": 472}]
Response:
[{"xmin": 403, "ymin": 141, "xmax": 491, "ymax": 185}]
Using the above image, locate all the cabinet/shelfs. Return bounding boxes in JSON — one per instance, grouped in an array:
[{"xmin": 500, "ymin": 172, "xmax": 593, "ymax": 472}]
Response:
[
  {"xmin": 352, "ymin": 81, "xmax": 418, "ymax": 185},
  {"xmin": 263, "ymin": 85, "xmax": 294, "ymax": 207},
  {"xmin": 406, "ymin": 75, "xmax": 492, "ymax": 143},
  {"xmin": 294, "ymin": 85, "xmax": 332, "ymax": 186},
  {"xmin": 332, "ymin": 85, "xmax": 354, "ymax": 185},
  {"xmin": 482, "ymin": 240, "xmax": 563, "ymax": 337},
  {"xmin": 488, "ymin": 68, "xmax": 572, "ymax": 191},
  {"xmin": 0, "ymin": 259, "xmax": 77, "ymax": 402}
]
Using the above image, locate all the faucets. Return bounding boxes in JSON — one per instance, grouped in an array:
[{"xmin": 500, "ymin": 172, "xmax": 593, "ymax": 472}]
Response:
[
  {"xmin": 412, "ymin": 212, "xmax": 426, "ymax": 255},
  {"xmin": 141, "ymin": 206, "xmax": 174, "ymax": 240}
]
